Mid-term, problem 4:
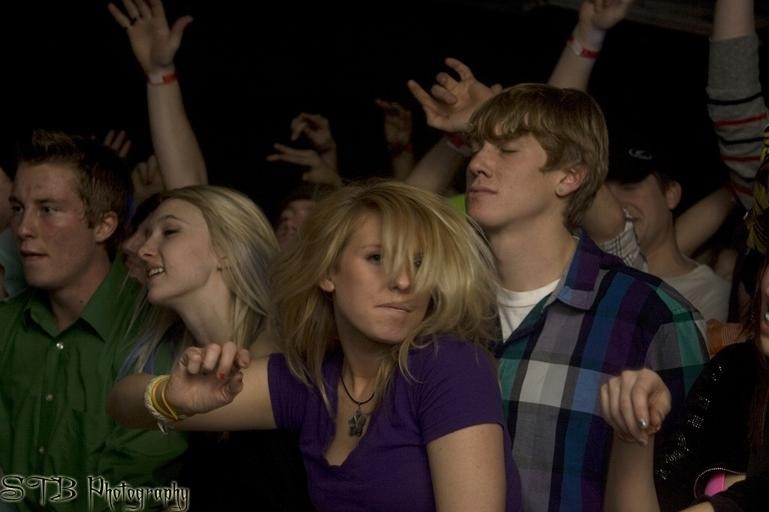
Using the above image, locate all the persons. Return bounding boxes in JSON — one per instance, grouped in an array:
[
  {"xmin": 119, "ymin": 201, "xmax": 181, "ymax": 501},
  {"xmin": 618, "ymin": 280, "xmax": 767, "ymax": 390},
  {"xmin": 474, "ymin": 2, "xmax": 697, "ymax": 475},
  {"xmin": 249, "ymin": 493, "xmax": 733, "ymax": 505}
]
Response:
[
  {"xmin": 2, "ymin": 132, "xmax": 191, "ymax": 511},
  {"xmin": 106, "ymin": 180, "xmax": 523, "ymax": 512},
  {"xmin": 135, "ymin": 183, "xmax": 281, "ymax": 359},
  {"xmin": 464, "ymin": 81, "xmax": 713, "ymax": 512},
  {"xmin": 597, "ymin": 259, "xmax": 769, "ymax": 511}
]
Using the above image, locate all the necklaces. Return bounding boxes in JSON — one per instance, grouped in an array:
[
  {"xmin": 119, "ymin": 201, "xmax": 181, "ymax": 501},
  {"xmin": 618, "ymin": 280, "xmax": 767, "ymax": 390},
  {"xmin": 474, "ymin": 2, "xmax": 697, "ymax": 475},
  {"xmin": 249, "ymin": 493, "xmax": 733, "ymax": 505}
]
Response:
[{"xmin": 340, "ymin": 375, "xmax": 379, "ymax": 432}]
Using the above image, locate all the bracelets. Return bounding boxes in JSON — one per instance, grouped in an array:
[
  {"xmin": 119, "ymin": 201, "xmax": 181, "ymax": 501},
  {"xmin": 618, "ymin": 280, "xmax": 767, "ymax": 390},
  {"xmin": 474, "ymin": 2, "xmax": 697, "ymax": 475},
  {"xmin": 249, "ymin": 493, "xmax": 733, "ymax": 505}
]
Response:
[{"xmin": 142, "ymin": 376, "xmax": 189, "ymax": 443}]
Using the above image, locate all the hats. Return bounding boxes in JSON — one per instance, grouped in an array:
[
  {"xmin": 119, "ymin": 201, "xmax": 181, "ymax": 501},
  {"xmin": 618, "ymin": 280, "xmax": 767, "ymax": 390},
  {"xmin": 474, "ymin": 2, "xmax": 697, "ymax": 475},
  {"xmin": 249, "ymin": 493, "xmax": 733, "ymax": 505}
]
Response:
[{"xmin": 601, "ymin": 132, "xmax": 675, "ymax": 185}]
